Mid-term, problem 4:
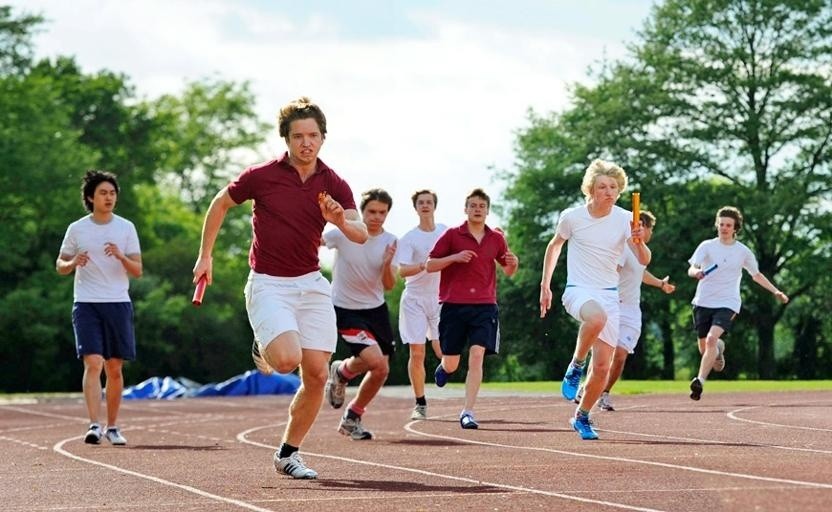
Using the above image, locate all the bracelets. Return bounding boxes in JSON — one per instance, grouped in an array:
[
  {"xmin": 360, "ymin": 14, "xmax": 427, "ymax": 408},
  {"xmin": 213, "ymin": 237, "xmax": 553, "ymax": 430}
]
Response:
[{"xmin": 661, "ymin": 280, "xmax": 665, "ymax": 289}]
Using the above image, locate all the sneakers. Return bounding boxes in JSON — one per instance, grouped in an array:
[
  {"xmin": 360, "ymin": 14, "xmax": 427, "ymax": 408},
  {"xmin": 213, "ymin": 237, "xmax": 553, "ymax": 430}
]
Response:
[
  {"xmin": 411, "ymin": 402, "xmax": 429, "ymax": 420},
  {"xmin": 459, "ymin": 412, "xmax": 479, "ymax": 428},
  {"xmin": 689, "ymin": 378, "xmax": 702, "ymax": 400},
  {"xmin": 272, "ymin": 450, "xmax": 319, "ymax": 480},
  {"xmin": 560, "ymin": 362, "xmax": 615, "ymax": 439},
  {"xmin": 337, "ymin": 415, "xmax": 373, "ymax": 440},
  {"xmin": 251, "ymin": 339, "xmax": 273, "ymax": 375},
  {"xmin": 712, "ymin": 338, "xmax": 725, "ymax": 372},
  {"xmin": 434, "ymin": 364, "xmax": 449, "ymax": 386},
  {"xmin": 84, "ymin": 423, "xmax": 103, "ymax": 442},
  {"xmin": 103, "ymin": 425, "xmax": 128, "ymax": 445},
  {"xmin": 325, "ymin": 359, "xmax": 348, "ymax": 409}
]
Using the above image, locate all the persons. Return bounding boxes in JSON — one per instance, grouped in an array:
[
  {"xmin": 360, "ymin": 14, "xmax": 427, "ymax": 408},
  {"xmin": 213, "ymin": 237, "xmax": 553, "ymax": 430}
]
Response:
[
  {"xmin": 54, "ymin": 169, "xmax": 145, "ymax": 445},
  {"xmin": 686, "ymin": 206, "xmax": 791, "ymax": 401},
  {"xmin": 425, "ymin": 189, "xmax": 519, "ymax": 430},
  {"xmin": 540, "ymin": 158, "xmax": 652, "ymax": 439},
  {"xmin": 575, "ymin": 211, "xmax": 678, "ymax": 412},
  {"xmin": 319, "ymin": 190, "xmax": 399, "ymax": 440},
  {"xmin": 393, "ymin": 191, "xmax": 503, "ymax": 419},
  {"xmin": 192, "ymin": 98, "xmax": 369, "ymax": 478}
]
